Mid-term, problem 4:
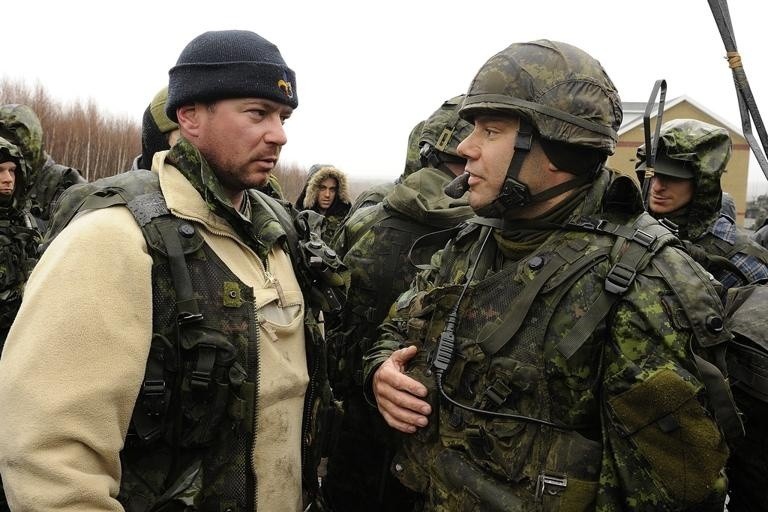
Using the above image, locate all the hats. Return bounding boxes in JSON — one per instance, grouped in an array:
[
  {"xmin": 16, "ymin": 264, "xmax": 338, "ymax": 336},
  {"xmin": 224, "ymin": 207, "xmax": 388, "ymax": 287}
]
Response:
[
  {"xmin": 634, "ymin": 158, "xmax": 695, "ymax": 179},
  {"xmin": 164, "ymin": 28, "xmax": 301, "ymax": 125}
]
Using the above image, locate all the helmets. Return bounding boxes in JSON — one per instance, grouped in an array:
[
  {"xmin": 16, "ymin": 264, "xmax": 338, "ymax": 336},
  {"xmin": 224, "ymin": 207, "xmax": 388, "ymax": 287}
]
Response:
[
  {"xmin": 0, "ymin": 138, "xmax": 20, "ymax": 159},
  {"xmin": 418, "ymin": 95, "xmax": 476, "ymax": 158},
  {"xmin": 406, "ymin": 120, "xmax": 428, "ymax": 165},
  {"xmin": 457, "ymin": 37, "xmax": 624, "ymax": 157},
  {"xmin": 149, "ymin": 87, "xmax": 180, "ymax": 133},
  {"xmin": 0, "ymin": 104, "xmax": 43, "ymax": 147}
]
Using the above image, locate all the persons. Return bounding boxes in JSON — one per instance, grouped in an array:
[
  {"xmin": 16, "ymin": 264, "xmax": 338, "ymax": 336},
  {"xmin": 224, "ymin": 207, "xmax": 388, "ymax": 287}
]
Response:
[
  {"xmin": 296, "ymin": 163, "xmax": 352, "ymax": 247},
  {"xmin": 632, "ymin": 115, "xmax": 739, "ymax": 278},
  {"xmin": 129, "ymin": 85, "xmax": 181, "ymax": 172},
  {"xmin": 353, "ymin": 36, "xmax": 744, "ymax": 512},
  {"xmin": 0, "ymin": 133, "xmax": 43, "ymax": 366},
  {"xmin": 329, "ymin": 95, "xmax": 479, "ymax": 512},
  {"xmin": 327, "ymin": 121, "xmax": 425, "ymax": 249},
  {"xmin": 0, "ymin": 101, "xmax": 88, "ymax": 244},
  {"xmin": 1, "ymin": 29, "xmax": 340, "ymax": 511}
]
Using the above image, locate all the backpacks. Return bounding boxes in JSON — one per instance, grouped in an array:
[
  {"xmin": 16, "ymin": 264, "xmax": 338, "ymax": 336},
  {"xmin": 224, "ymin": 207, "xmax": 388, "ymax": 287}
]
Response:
[{"xmin": 722, "ymin": 284, "xmax": 768, "ymax": 410}]
